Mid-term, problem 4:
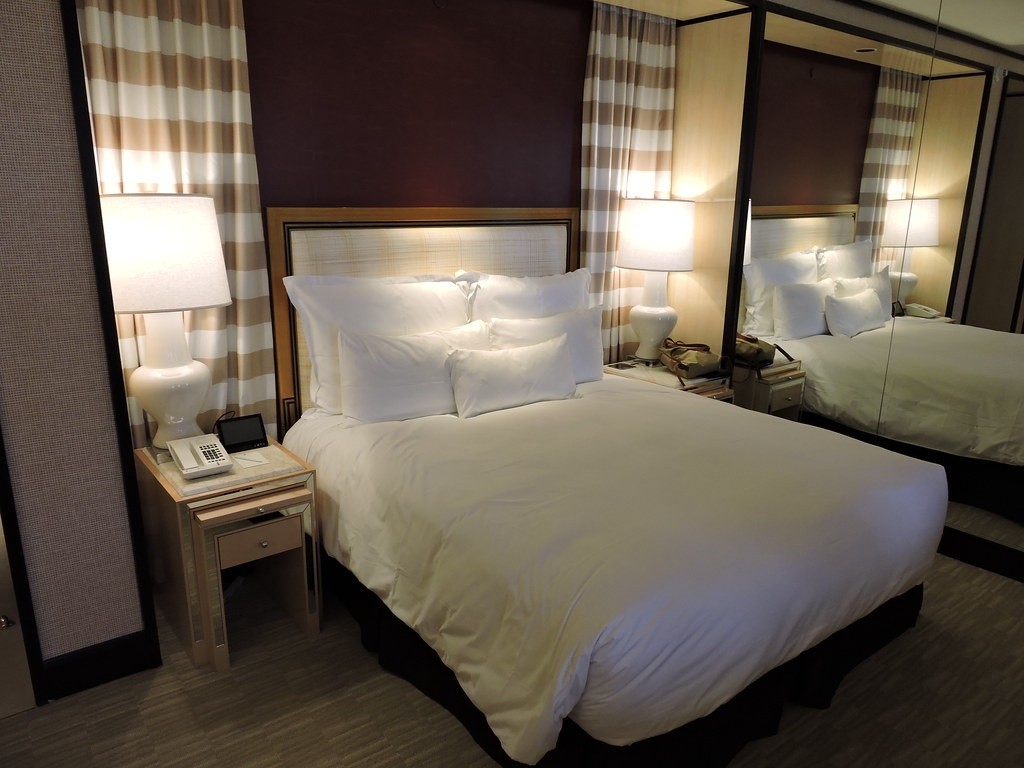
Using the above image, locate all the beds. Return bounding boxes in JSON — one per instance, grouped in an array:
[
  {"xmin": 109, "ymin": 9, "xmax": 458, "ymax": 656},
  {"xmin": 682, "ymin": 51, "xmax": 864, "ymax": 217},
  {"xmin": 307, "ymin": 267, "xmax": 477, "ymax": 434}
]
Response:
[
  {"xmin": 754, "ymin": 315, "xmax": 1024, "ymax": 469},
  {"xmin": 279, "ymin": 370, "xmax": 949, "ymax": 747}
]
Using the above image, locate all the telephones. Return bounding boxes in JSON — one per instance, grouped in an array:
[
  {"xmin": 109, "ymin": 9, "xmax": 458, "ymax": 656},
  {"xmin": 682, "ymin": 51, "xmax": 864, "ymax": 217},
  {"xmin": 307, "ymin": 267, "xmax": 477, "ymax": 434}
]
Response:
[
  {"xmin": 165, "ymin": 433, "xmax": 234, "ymax": 480},
  {"xmin": 905, "ymin": 302, "xmax": 941, "ymax": 318}
]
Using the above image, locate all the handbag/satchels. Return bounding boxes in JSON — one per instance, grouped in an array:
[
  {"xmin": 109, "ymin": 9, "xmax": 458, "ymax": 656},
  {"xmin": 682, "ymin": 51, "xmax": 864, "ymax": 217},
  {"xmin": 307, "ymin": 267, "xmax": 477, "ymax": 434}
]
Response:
[
  {"xmin": 657, "ymin": 338, "xmax": 721, "ymax": 387},
  {"xmin": 733, "ymin": 332, "xmax": 795, "ymax": 381}
]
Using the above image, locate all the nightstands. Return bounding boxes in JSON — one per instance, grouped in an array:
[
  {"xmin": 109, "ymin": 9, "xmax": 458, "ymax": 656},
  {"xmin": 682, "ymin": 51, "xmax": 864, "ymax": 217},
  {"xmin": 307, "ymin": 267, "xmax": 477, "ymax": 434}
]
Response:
[
  {"xmin": 134, "ymin": 433, "xmax": 322, "ymax": 674},
  {"xmin": 934, "ymin": 315, "xmax": 956, "ymax": 325},
  {"xmin": 733, "ymin": 358, "xmax": 806, "ymax": 422},
  {"xmin": 604, "ymin": 358, "xmax": 735, "ymax": 405}
]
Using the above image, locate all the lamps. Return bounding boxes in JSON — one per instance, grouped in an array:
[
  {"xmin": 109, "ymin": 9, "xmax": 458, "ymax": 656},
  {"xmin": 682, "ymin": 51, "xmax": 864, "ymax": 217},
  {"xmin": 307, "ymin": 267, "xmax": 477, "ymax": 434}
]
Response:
[
  {"xmin": 100, "ymin": 193, "xmax": 234, "ymax": 450},
  {"xmin": 615, "ymin": 198, "xmax": 696, "ymax": 362},
  {"xmin": 878, "ymin": 197, "xmax": 940, "ymax": 310}
]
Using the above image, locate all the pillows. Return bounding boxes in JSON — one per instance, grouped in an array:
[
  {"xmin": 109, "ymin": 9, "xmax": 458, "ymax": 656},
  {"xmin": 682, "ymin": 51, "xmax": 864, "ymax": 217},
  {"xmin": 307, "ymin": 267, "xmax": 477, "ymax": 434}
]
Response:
[
  {"xmin": 282, "ymin": 268, "xmax": 481, "ymax": 419},
  {"xmin": 824, "ymin": 288, "xmax": 886, "ymax": 339},
  {"xmin": 833, "ymin": 264, "xmax": 893, "ymax": 322},
  {"xmin": 444, "ymin": 332, "xmax": 583, "ymax": 419},
  {"xmin": 741, "ymin": 245, "xmax": 819, "ymax": 339},
  {"xmin": 813, "ymin": 238, "xmax": 872, "ymax": 282},
  {"xmin": 337, "ymin": 319, "xmax": 488, "ymax": 428},
  {"xmin": 454, "ymin": 267, "xmax": 592, "ymax": 322},
  {"xmin": 771, "ymin": 277, "xmax": 834, "ymax": 344},
  {"xmin": 488, "ymin": 304, "xmax": 605, "ymax": 384}
]
